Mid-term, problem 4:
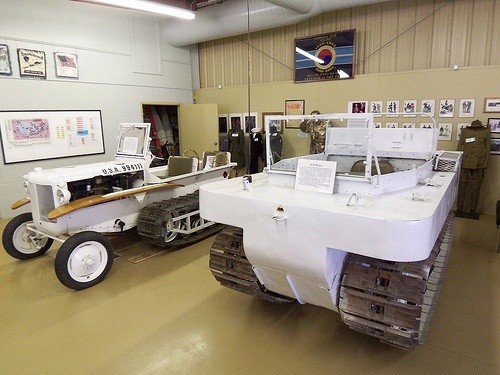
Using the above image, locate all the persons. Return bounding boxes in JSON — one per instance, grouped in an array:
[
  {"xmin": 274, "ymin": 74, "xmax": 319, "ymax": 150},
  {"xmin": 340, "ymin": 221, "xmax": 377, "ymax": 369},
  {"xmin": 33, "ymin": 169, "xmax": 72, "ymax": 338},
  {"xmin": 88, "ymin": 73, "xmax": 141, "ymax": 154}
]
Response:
[
  {"xmin": 261, "ymin": 126, "xmax": 282, "ymax": 166},
  {"xmin": 300, "ymin": 110, "xmax": 334, "ymax": 154},
  {"xmin": 247, "ymin": 128, "xmax": 263, "ymax": 174},
  {"xmin": 456, "ymin": 120, "xmax": 492, "ymax": 217},
  {"xmin": 228, "ymin": 121, "xmax": 246, "ymax": 169}
]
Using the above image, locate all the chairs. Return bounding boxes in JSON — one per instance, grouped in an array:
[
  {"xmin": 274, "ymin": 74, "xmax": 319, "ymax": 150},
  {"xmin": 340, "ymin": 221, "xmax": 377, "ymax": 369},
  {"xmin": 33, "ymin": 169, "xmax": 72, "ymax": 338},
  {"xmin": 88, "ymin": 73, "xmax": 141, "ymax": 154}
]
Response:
[
  {"xmin": 350, "ymin": 159, "xmax": 398, "ymax": 177},
  {"xmin": 158, "ymin": 156, "xmax": 194, "ymax": 180},
  {"xmin": 202, "ymin": 151, "xmax": 221, "ymax": 170}
]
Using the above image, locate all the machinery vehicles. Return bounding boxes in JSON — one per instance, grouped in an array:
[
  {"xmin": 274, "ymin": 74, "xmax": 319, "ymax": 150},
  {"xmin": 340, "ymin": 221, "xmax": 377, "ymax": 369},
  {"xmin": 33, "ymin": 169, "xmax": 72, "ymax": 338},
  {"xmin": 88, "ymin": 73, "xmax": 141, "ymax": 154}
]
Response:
[
  {"xmin": 2, "ymin": 119, "xmax": 232, "ymax": 292},
  {"xmin": 199, "ymin": 115, "xmax": 468, "ymax": 347}
]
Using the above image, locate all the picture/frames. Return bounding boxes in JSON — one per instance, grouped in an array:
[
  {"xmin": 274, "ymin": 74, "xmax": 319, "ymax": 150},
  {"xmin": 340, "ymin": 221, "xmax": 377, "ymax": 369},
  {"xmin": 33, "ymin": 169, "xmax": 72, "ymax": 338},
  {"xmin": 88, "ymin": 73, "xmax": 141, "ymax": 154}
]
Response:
[
  {"xmin": 292, "ymin": 29, "xmax": 355, "ymax": 84},
  {"xmin": 285, "ymin": 100, "xmax": 305, "ymax": 129},
  {"xmin": 351, "ymin": 97, "xmax": 500, "ymax": 155},
  {"xmin": 0, "ymin": 110, "xmax": 106, "ymax": 164},
  {"xmin": 261, "ymin": 112, "xmax": 284, "ymax": 134},
  {"xmin": 1, "ymin": 44, "xmax": 79, "ymax": 78}
]
